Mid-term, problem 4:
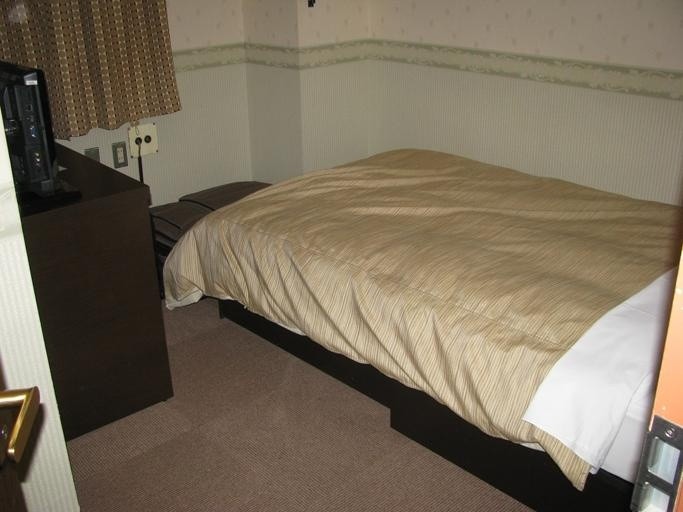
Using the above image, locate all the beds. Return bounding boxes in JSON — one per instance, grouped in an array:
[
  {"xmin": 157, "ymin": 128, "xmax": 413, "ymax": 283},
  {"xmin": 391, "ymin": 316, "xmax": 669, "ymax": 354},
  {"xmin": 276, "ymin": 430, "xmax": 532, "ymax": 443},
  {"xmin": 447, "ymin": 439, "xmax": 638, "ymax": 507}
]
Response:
[{"xmin": 210, "ymin": 144, "xmax": 683, "ymax": 512}]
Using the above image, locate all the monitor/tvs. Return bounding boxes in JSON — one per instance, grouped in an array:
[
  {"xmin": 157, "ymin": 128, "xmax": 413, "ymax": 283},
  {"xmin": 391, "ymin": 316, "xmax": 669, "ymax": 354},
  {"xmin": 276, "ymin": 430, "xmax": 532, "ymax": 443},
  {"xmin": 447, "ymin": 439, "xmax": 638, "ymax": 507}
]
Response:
[{"xmin": 0, "ymin": 60, "xmax": 62, "ymax": 198}]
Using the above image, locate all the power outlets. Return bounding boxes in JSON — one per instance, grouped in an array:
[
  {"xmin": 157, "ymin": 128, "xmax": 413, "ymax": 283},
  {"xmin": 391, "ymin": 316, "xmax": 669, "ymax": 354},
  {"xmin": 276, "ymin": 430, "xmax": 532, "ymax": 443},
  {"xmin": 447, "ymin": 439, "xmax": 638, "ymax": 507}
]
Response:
[{"xmin": 111, "ymin": 140, "xmax": 130, "ymax": 169}]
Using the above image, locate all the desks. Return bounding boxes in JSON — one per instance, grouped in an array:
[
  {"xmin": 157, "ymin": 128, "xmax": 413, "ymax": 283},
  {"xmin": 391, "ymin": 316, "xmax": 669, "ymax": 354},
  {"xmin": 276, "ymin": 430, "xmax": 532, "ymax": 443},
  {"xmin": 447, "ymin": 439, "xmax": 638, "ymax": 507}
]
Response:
[{"xmin": 10, "ymin": 140, "xmax": 176, "ymax": 447}]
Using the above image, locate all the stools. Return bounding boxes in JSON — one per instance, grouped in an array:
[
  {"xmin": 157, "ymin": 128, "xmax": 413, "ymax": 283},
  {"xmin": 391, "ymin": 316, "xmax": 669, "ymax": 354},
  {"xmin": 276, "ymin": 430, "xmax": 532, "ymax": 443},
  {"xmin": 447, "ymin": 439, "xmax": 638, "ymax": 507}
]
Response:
[{"xmin": 149, "ymin": 180, "xmax": 274, "ymax": 300}]
